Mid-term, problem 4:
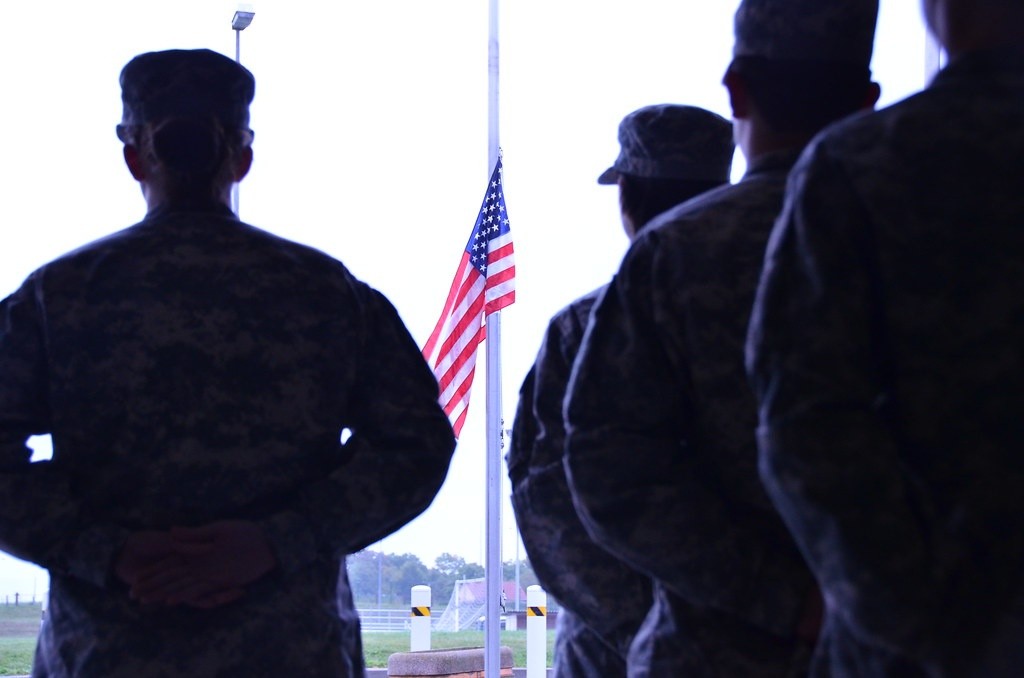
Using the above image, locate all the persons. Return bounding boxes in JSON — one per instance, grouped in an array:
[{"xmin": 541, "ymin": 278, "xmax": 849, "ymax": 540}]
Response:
[
  {"xmin": 562, "ymin": 0, "xmax": 889, "ymax": 678},
  {"xmin": 505, "ymin": 104, "xmax": 735, "ymax": 678},
  {"xmin": 0, "ymin": 48, "xmax": 456, "ymax": 678},
  {"xmin": 746, "ymin": 0, "xmax": 1024, "ymax": 678}
]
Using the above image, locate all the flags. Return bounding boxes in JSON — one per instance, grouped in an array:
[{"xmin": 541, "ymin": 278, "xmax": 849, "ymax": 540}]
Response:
[{"xmin": 422, "ymin": 158, "xmax": 517, "ymax": 440}]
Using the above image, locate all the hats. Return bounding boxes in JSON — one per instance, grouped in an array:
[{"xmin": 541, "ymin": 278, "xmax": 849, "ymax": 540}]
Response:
[
  {"xmin": 117, "ymin": 48, "xmax": 254, "ymax": 145},
  {"xmin": 599, "ymin": 104, "xmax": 735, "ymax": 188},
  {"xmin": 734, "ymin": 0, "xmax": 879, "ymax": 76}
]
